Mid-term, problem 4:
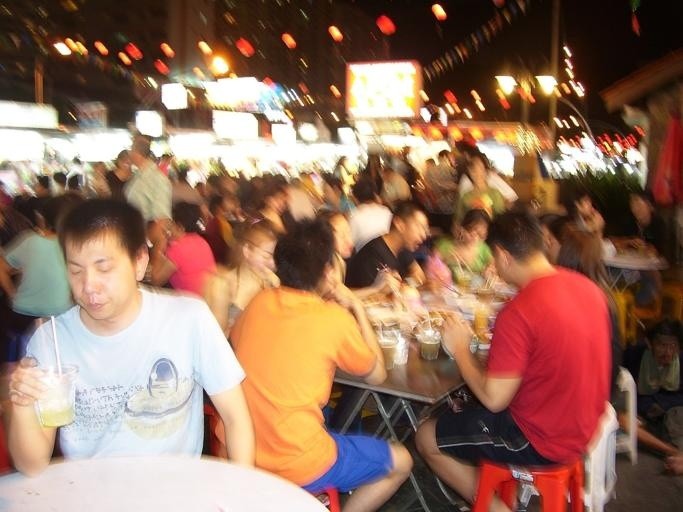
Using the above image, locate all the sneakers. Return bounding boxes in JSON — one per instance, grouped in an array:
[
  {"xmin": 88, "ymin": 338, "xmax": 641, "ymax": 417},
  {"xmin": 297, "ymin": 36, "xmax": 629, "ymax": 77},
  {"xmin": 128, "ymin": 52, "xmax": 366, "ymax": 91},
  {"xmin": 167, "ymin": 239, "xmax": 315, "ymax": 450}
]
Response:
[{"xmin": 663, "ymin": 454, "xmax": 683, "ymax": 476}]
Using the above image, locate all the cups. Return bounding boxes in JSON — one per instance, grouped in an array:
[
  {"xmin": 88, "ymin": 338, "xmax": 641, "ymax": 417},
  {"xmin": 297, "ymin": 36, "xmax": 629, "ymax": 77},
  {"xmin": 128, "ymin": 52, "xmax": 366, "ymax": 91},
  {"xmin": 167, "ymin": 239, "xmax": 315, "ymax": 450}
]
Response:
[
  {"xmin": 419, "ymin": 329, "xmax": 442, "ymax": 360},
  {"xmin": 376, "ymin": 338, "xmax": 396, "ymax": 370},
  {"xmin": 30, "ymin": 365, "xmax": 79, "ymax": 428}
]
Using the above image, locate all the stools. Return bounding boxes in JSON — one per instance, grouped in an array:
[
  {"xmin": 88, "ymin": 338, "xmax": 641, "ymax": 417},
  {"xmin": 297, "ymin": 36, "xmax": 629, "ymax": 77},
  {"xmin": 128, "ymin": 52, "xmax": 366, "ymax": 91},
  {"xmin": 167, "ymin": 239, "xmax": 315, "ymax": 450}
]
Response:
[{"xmin": 205, "ymin": 281, "xmax": 682, "ymax": 512}]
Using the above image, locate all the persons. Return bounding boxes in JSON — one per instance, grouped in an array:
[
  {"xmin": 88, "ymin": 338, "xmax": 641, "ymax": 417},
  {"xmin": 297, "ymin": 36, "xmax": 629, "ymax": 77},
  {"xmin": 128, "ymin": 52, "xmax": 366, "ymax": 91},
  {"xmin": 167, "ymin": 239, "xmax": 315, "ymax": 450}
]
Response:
[{"xmin": 0, "ymin": 132, "xmax": 683, "ymax": 511}]
